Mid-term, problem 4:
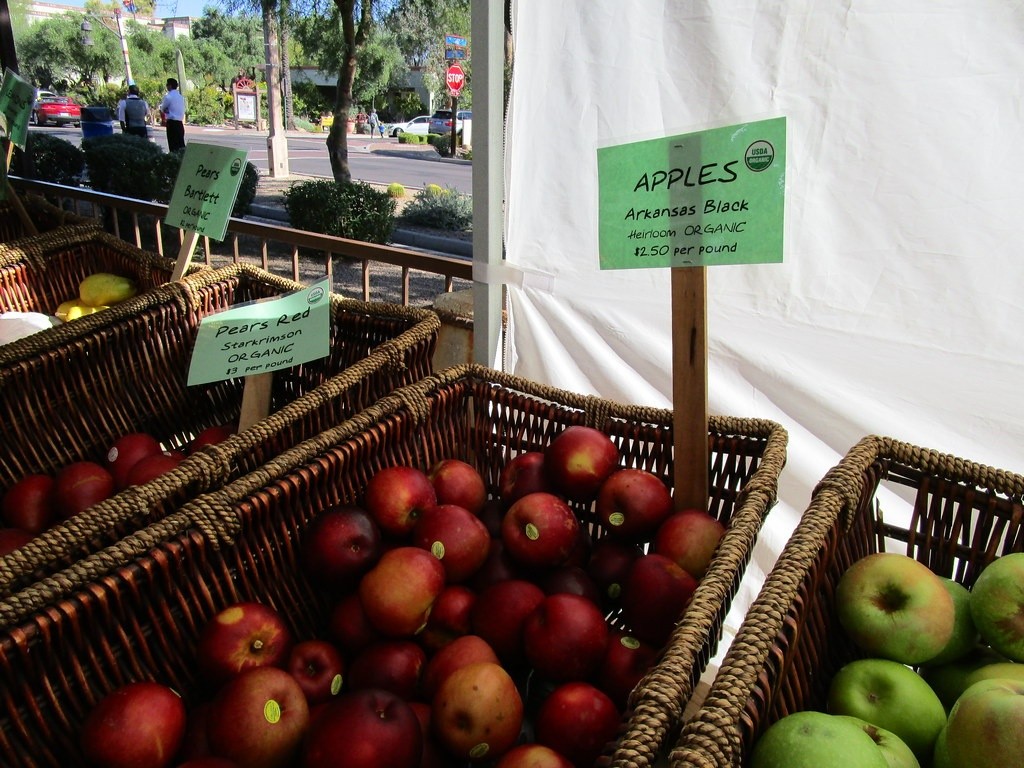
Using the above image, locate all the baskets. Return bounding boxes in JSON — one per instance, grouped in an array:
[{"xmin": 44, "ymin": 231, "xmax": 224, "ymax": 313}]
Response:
[{"xmin": 0, "ymin": 194, "xmax": 1024, "ymax": 768}]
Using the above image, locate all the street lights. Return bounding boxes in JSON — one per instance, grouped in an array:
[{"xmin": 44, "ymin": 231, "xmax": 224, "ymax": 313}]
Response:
[{"xmin": 80, "ymin": 8, "xmax": 135, "ymax": 85}]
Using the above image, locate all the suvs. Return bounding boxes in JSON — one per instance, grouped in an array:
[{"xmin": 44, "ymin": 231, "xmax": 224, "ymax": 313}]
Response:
[{"xmin": 428, "ymin": 109, "xmax": 473, "ymax": 137}]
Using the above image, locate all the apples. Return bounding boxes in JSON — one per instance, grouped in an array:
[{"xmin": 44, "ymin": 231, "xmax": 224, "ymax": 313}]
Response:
[
  {"xmin": 0, "ymin": 429, "xmax": 237, "ymax": 557},
  {"xmin": 86, "ymin": 427, "xmax": 725, "ymax": 768},
  {"xmin": 753, "ymin": 553, "xmax": 1024, "ymax": 768}
]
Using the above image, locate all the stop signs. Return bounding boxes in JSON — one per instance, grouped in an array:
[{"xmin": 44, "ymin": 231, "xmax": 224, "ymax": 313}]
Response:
[{"xmin": 446, "ymin": 65, "xmax": 465, "ymax": 91}]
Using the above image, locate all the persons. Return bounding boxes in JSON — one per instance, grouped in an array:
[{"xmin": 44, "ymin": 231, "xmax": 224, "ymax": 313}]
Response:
[
  {"xmin": 377, "ymin": 123, "xmax": 385, "ymax": 138},
  {"xmin": 119, "ymin": 85, "xmax": 150, "ymax": 138},
  {"xmin": 161, "ymin": 78, "xmax": 185, "ymax": 152},
  {"xmin": 369, "ymin": 108, "xmax": 378, "ymax": 138}
]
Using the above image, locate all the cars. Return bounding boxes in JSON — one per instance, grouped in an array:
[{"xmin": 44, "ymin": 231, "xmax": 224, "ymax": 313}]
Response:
[
  {"xmin": 387, "ymin": 115, "xmax": 432, "ymax": 138},
  {"xmin": 28, "ymin": 90, "xmax": 82, "ymax": 128}
]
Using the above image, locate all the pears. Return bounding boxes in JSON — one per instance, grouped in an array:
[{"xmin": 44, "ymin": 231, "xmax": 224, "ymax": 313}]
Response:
[{"xmin": 56, "ymin": 274, "xmax": 135, "ymax": 321}]
